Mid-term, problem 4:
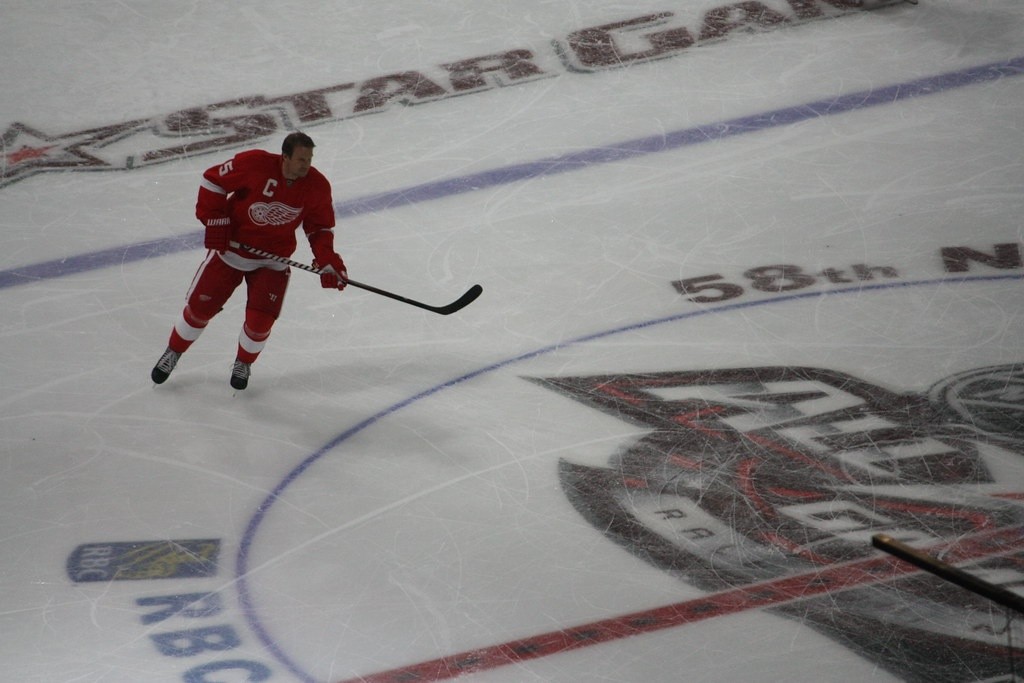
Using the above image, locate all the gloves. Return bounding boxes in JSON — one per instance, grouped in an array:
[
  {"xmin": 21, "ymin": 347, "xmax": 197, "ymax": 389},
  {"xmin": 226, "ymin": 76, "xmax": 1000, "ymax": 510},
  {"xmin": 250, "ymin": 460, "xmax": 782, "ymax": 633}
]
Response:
[
  {"xmin": 204, "ymin": 213, "xmax": 231, "ymax": 255},
  {"xmin": 321, "ymin": 261, "xmax": 348, "ymax": 290}
]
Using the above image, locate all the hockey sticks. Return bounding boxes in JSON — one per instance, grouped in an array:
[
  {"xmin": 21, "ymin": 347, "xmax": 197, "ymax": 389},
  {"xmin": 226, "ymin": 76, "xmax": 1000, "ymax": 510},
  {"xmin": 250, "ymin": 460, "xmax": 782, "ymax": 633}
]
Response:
[
  {"xmin": 869, "ymin": 533, "xmax": 1024, "ymax": 614},
  {"xmin": 227, "ymin": 237, "xmax": 484, "ymax": 317}
]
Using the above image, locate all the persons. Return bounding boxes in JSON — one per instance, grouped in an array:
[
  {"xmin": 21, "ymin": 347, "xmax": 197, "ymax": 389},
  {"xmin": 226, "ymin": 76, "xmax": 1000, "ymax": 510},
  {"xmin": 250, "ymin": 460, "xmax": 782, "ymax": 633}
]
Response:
[{"xmin": 151, "ymin": 131, "xmax": 349, "ymax": 397}]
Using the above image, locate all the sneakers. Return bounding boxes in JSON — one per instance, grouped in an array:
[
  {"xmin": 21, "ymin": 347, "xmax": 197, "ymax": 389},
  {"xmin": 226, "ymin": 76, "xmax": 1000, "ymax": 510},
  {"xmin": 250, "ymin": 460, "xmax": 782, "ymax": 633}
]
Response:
[
  {"xmin": 151, "ymin": 346, "xmax": 181, "ymax": 389},
  {"xmin": 229, "ymin": 358, "xmax": 251, "ymax": 397}
]
